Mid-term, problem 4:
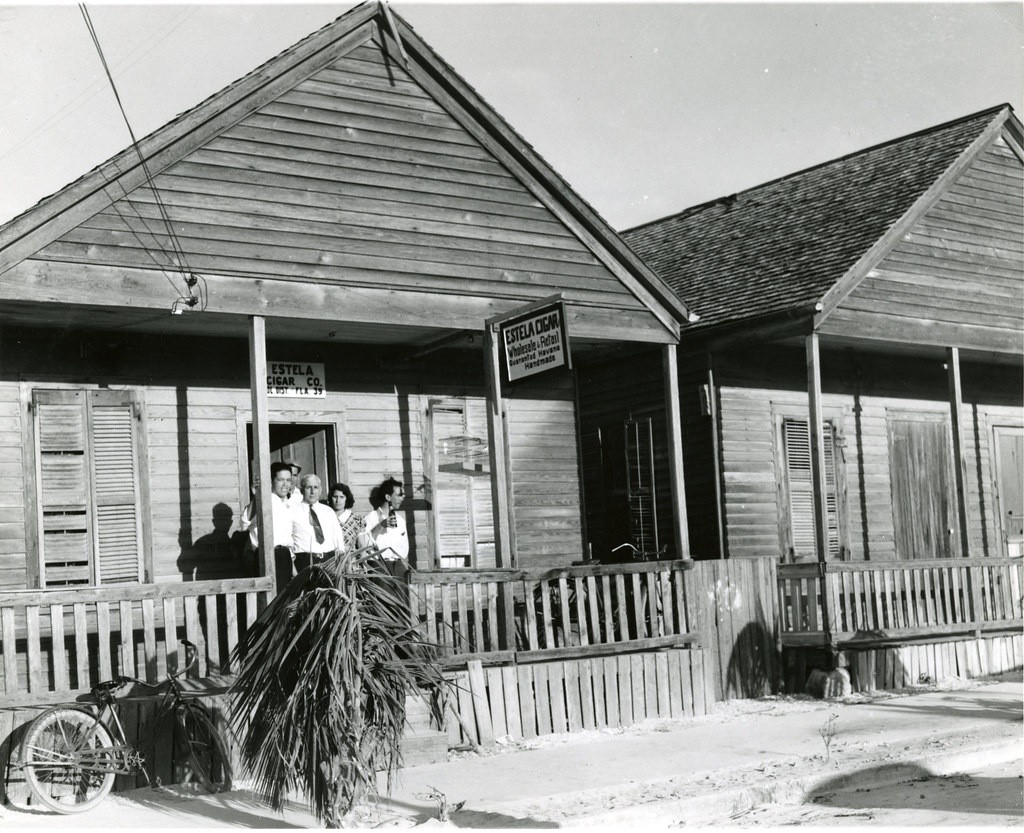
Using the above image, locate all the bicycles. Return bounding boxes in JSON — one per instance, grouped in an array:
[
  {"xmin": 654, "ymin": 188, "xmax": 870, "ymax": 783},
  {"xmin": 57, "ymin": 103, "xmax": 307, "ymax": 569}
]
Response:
[
  {"xmin": 12, "ymin": 640, "xmax": 234, "ymax": 815},
  {"xmin": 521, "ymin": 543, "xmax": 674, "ymax": 662}
]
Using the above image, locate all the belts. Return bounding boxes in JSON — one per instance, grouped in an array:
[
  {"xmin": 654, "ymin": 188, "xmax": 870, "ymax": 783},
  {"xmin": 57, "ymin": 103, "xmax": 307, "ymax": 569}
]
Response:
[
  {"xmin": 275, "ymin": 545, "xmax": 287, "ymax": 549},
  {"xmin": 296, "ymin": 552, "xmax": 332, "ymax": 559}
]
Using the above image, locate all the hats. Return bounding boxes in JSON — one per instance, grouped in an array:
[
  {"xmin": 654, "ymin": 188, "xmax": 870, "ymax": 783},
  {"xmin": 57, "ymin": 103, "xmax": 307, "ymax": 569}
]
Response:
[{"xmin": 287, "ymin": 462, "xmax": 302, "ymax": 473}]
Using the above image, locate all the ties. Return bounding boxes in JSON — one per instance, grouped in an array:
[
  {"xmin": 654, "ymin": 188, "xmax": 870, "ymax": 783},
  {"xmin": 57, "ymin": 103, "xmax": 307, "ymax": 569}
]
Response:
[
  {"xmin": 286, "ymin": 492, "xmax": 291, "ymax": 498},
  {"xmin": 310, "ymin": 504, "xmax": 324, "ymax": 545}
]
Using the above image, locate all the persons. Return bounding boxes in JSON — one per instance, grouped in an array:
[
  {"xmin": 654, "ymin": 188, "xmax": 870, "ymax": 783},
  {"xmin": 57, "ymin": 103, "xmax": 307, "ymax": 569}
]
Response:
[{"xmin": 239, "ymin": 461, "xmax": 411, "ymax": 660}]
